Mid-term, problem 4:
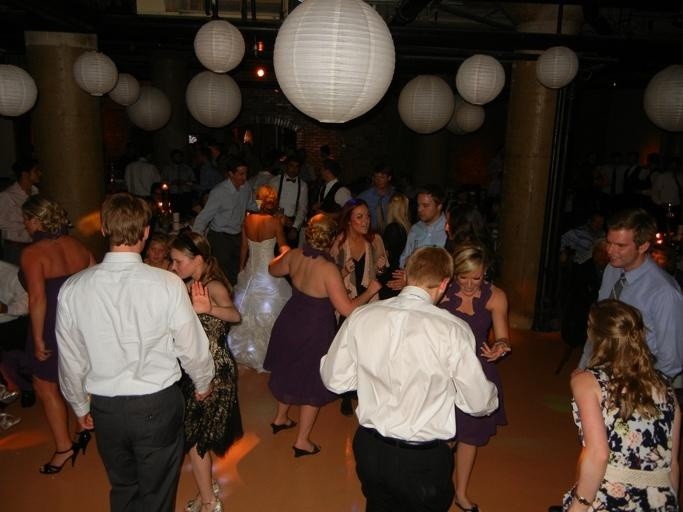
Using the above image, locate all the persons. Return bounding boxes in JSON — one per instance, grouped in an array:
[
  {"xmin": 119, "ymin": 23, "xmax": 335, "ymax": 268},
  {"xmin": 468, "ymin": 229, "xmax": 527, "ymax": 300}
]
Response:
[
  {"xmin": 125, "ymin": 148, "xmax": 161, "ymax": 197},
  {"xmin": 321, "ymin": 246, "xmax": 498, "ymax": 512},
  {"xmin": 227, "ymin": 185, "xmax": 285, "ymax": 369},
  {"xmin": 560, "ymin": 209, "xmax": 607, "ymax": 284},
  {"xmin": 377, "ymin": 196, "xmax": 407, "ymax": 297},
  {"xmin": 268, "ymin": 214, "xmax": 381, "ymax": 457},
  {"xmin": 192, "ymin": 189, "xmax": 209, "ymax": 220},
  {"xmin": 399, "ymin": 190, "xmax": 448, "ymax": 269},
  {"xmin": 19, "ymin": 194, "xmax": 96, "ymax": 474},
  {"xmin": 312, "ymin": 160, "xmax": 352, "ymax": 216},
  {"xmin": 268, "ymin": 157, "xmax": 309, "ymax": 246},
  {"xmin": 445, "ymin": 205, "xmax": 487, "ymax": 256},
  {"xmin": 601, "ymin": 208, "xmax": 682, "ymax": 387},
  {"xmin": 0, "ymin": 155, "xmax": 42, "ymax": 261},
  {"xmin": 564, "ymin": 300, "xmax": 680, "ymax": 512},
  {"xmin": 150, "ymin": 182, "xmax": 167, "ymax": 217},
  {"xmin": 0, "ymin": 261, "xmax": 36, "ymax": 407},
  {"xmin": 438, "ymin": 245, "xmax": 510, "ymax": 510},
  {"xmin": 355, "ymin": 163, "xmax": 396, "ymax": 229},
  {"xmin": 330, "ymin": 198, "xmax": 388, "ymax": 416},
  {"xmin": 56, "ymin": 195, "xmax": 213, "ymax": 510},
  {"xmin": 171, "ymin": 235, "xmax": 242, "ymax": 510},
  {"xmin": 192, "ymin": 161, "xmax": 256, "ymax": 284}
]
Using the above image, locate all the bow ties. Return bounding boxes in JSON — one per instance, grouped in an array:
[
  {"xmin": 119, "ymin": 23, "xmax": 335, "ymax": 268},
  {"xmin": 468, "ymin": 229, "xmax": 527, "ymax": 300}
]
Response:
[{"xmin": 286, "ymin": 177, "xmax": 297, "ymax": 183}]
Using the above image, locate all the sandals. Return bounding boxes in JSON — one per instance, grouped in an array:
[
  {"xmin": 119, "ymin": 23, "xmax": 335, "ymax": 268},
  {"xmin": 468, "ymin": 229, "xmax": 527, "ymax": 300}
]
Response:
[
  {"xmin": 0, "ymin": 383, "xmax": 19, "ymax": 402},
  {"xmin": 0, "ymin": 413, "xmax": 23, "ymax": 431}
]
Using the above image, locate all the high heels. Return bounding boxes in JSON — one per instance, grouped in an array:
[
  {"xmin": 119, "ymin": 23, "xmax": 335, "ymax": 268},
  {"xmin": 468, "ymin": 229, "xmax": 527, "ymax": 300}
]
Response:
[
  {"xmin": 292, "ymin": 442, "xmax": 322, "ymax": 458},
  {"xmin": 38, "ymin": 440, "xmax": 80, "ymax": 474},
  {"xmin": 185, "ymin": 478, "xmax": 220, "ymax": 512},
  {"xmin": 214, "ymin": 495, "xmax": 223, "ymax": 512},
  {"xmin": 70, "ymin": 430, "xmax": 89, "ymax": 455},
  {"xmin": 271, "ymin": 418, "xmax": 297, "ymax": 433}
]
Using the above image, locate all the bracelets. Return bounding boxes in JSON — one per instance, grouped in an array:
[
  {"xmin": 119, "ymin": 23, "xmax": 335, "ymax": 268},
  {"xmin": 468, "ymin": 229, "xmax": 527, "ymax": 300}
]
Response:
[
  {"xmin": 571, "ymin": 486, "xmax": 593, "ymax": 507},
  {"xmin": 495, "ymin": 340, "xmax": 506, "ymax": 356}
]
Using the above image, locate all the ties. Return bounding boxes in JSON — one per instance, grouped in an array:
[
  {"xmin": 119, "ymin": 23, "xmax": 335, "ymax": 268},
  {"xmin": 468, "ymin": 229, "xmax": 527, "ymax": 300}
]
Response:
[
  {"xmin": 609, "ymin": 272, "xmax": 627, "ymax": 300},
  {"xmin": 319, "ymin": 185, "xmax": 326, "ymax": 202},
  {"xmin": 374, "ymin": 195, "xmax": 385, "ymax": 230}
]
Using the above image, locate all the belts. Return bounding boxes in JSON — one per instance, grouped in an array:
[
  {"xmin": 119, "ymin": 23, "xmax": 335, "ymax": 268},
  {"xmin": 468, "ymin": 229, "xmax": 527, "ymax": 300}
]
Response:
[{"xmin": 363, "ymin": 427, "xmax": 442, "ymax": 449}]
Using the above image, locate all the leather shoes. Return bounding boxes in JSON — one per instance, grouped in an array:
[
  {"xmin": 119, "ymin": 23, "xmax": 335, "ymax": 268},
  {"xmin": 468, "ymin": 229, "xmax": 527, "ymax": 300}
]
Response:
[{"xmin": 454, "ymin": 498, "xmax": 481, "ymax": 512}]
